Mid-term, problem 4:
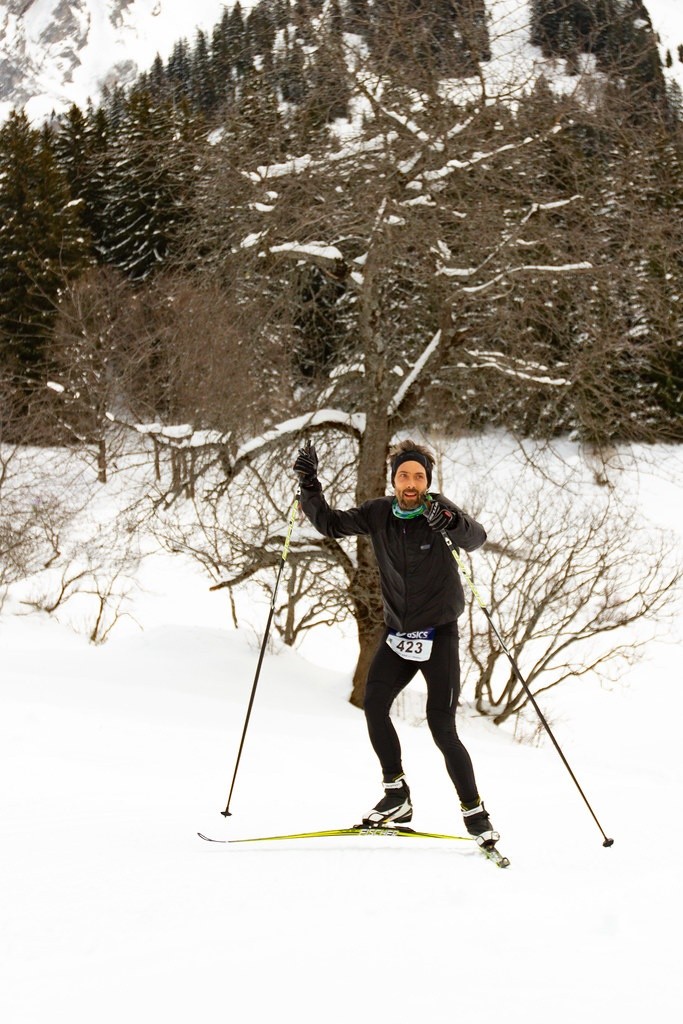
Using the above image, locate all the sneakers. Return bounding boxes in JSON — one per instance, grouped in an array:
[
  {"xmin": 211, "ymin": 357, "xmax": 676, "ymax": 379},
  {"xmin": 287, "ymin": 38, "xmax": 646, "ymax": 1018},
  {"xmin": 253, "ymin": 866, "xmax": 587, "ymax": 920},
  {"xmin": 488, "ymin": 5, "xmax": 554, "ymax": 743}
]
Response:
[
  {"xmin": 459, "ymin": 797, "xmax": 499, "ymax": 847},
  {"xmin": 362, "ymin": 772, "xmax": 413, "ymax": 824}
]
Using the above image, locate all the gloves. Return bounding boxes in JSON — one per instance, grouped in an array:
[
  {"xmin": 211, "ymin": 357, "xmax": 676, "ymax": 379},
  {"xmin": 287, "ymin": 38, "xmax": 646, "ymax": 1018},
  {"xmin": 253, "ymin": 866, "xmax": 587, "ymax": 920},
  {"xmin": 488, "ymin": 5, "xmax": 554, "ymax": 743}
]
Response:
[
  {"xmin": 292, "ymin": 446, "xmax": 318, "ymax": 486},
  {"xmin": 423, "ymin": 500, "xmax": 456, "ymax": 532}
]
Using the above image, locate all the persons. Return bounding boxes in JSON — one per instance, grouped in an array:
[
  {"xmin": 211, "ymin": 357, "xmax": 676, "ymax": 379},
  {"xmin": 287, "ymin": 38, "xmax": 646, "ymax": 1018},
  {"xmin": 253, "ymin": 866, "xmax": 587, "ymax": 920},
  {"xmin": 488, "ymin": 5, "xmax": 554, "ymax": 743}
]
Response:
[{"xmin": 293, "ymin": 442, "xmax": 500, "ymax": 846}]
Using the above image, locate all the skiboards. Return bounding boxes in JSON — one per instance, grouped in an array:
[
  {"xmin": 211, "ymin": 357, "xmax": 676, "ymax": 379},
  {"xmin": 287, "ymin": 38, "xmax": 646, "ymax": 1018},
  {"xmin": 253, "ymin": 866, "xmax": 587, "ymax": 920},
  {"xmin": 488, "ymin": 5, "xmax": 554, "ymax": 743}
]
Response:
[{"xmin": 196, "ymin": 824, "xmax": 512, "ymax": 870}]
